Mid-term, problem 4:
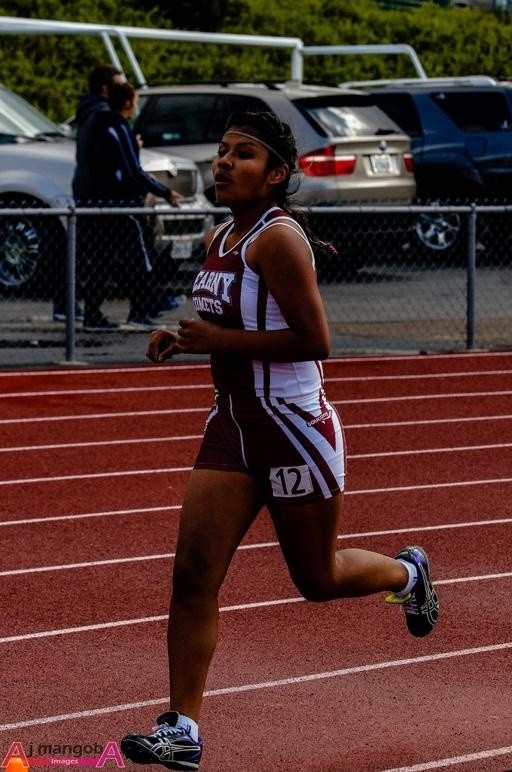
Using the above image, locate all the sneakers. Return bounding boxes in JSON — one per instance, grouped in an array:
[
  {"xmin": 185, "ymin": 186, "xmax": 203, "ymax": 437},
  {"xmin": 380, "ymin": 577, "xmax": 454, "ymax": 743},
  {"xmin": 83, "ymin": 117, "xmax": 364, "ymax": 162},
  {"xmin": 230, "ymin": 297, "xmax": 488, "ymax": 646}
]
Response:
[
  {"xmin": 385, "ymin": 546, "xmax": 439, "ymax": 637},
  {"xmin": 129, "ymin": 316, "xmax": 166, "ymax": 332},
  {"xmin": 120, "ymin": 710, "xmax": 203, "ymax": 771},
  {"xmin": 52, "ymin": 306, "xmax": 87, "ymax": 320},
  {"xmin": 83, "ymin": 311, "xmax": 123, "ymax": 332}
]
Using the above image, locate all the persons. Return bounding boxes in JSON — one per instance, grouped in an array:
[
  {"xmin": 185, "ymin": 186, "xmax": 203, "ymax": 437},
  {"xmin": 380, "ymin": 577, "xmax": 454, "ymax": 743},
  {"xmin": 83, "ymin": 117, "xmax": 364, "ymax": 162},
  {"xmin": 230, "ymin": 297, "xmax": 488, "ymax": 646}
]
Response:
[
  {"xmin": 52, "ymin": 62, "xmax": 184, "ymax": 332},
  {"xmin": 120, "ymin": 109, "xmax": 441, "ymax": 772}
]
